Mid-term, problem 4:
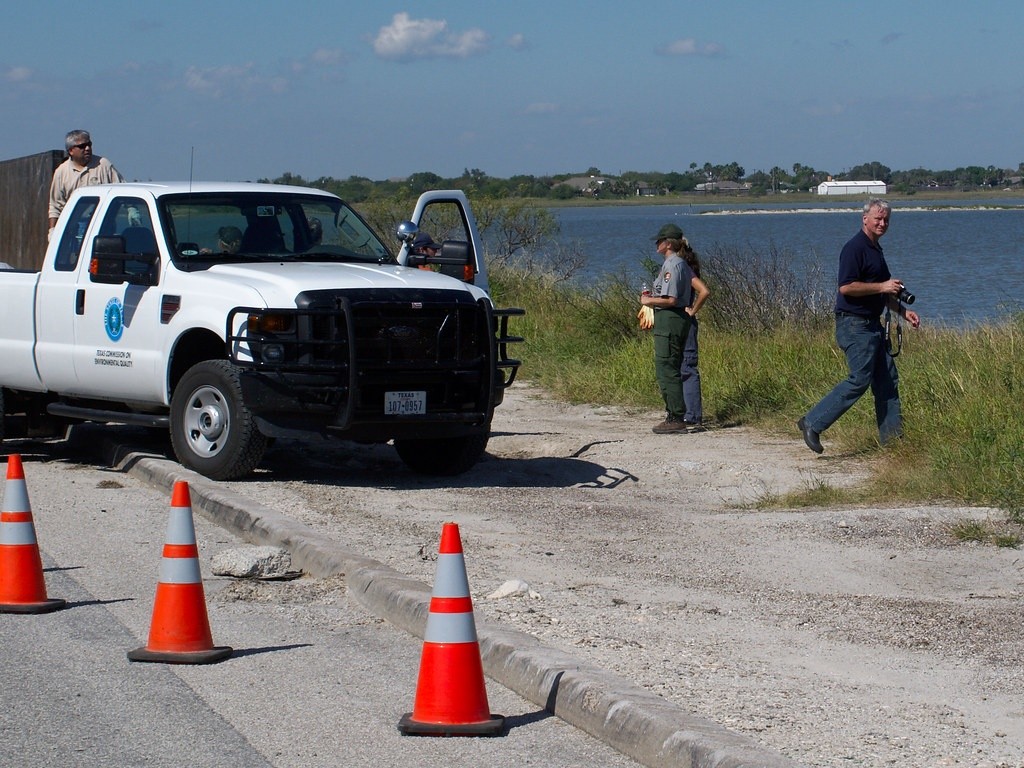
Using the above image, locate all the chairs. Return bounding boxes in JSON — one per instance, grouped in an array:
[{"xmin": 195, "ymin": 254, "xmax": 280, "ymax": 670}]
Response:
[
  {"xmin": 239, "ymin": 204, "xmax": 316, "ymax": 254},
  {"xmin": 122, "ymin": 225, "xmax": 161, "ymax": 275}
]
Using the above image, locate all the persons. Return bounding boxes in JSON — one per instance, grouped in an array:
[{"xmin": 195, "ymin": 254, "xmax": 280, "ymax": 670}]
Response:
[
  {"xmin": 796, "ymin": 196, "xmax": 920, "ymax": 453},
  {"xmin": 306, "ymin": 217, "xmax": 323, "ymax": 241},
  {"xmin": 676, "ymin": 238, "xmax": 710, "ymax": 426},
  {"xmin": 412, "ymin": 232, "xmax": 442, "ymax": 272},
  {"xmin": 641, "ymin": 224, "xmax": 692, "ymax": 434},
  {"xmin": 48, "ymin": 130, "xmax": 143, "ymax": 243},
  {"xmin": 200, "ymin": 227, "xmax": 243, "ymax": 254}
]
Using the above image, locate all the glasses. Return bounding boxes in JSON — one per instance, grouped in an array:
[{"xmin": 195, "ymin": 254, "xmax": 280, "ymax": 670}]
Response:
[{"xmin": 74, "ymin": 140, "xmax": 92, "ymax": 149}]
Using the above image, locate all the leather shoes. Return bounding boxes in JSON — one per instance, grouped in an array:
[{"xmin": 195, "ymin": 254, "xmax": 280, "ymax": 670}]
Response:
[{"xmin": 798, "ymin": 416, "xmax": 823, "ymax": 454}]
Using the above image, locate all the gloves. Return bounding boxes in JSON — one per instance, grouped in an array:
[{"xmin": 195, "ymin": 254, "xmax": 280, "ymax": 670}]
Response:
[
  {"xmin": 127, "ymin": 207, "xmax": 143, "ymax": 226},
  {"xmin": 47, "ymin": 227, "xmax": 54, "ymax": 243}
]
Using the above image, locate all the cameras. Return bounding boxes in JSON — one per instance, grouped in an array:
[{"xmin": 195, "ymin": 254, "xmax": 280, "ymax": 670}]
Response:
[{"xmin": 881, "ymin": 281, "xmax": 915, "ymax": 305}]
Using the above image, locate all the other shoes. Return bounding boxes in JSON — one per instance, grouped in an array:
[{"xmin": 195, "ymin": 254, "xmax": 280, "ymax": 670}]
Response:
[{"xmin": 683, "ymin": 418, "xmax": 700, "ymax": 427}]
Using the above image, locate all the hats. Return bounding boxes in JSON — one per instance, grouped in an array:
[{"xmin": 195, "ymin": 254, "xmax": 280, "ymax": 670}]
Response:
[
  {"xmin": 412, "ymin": 233, "xmax": 442, "ymax": 249},
  {"xmin": 649, "ymin": 224, "xmax": 683, "ymax": 240},
  {"xmin": 219, "ymin": 226, "xmax": 242, "ymax": 246}
]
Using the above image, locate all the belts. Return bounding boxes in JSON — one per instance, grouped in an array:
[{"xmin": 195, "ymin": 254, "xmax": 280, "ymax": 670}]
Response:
[{"xmin": 836, "ymin": 311, "xmax": 859, "ymax": 317}]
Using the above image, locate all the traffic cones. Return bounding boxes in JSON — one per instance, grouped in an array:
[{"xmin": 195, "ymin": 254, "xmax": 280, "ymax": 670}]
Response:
[
  {"xmin": 1, "ymin": 453, "xmax": 69, "ymax": 614},
  {"xmin": 396, "ymin": 520, "xmax": 507, "ymax": 736},
  {"xmin": 124, "ymin": 479, "xmax": 235, "ymax": 665}
]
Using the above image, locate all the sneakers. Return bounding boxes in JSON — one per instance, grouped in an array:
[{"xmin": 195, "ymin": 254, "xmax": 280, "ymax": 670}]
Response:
[{"xmin": 652, "ymin": 418, "xmax": 688, "ymax": 434}]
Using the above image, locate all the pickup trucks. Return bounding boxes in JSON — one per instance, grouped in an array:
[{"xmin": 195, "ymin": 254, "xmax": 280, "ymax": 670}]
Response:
[{"xmin": 1, "ymin": 180, "xmax": 526, "ymax": 483}]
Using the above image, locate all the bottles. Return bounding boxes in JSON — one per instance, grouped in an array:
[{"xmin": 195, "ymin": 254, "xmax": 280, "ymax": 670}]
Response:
[{"xmin": 642, "ymin": 283, "xmax": 652, "ymax": 298}]
